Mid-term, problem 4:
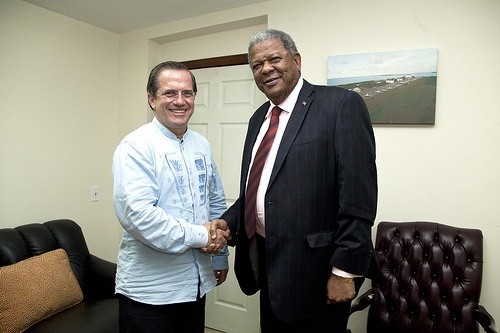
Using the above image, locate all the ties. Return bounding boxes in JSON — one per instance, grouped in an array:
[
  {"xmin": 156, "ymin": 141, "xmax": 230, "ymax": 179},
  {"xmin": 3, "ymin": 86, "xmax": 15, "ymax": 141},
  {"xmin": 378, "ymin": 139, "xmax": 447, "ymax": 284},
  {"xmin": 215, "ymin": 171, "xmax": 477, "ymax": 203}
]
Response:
[{"xmin": 244, "ymin": 105, "xmax": 282, "ymax": 240}]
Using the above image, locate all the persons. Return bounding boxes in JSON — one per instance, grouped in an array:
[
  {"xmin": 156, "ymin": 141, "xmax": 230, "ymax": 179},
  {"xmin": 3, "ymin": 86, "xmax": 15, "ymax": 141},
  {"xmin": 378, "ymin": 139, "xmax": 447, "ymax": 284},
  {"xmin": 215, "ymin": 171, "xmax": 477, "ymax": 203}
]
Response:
[
  {"xmin": 114, "ymin": 61, "xmax": 230, "ymax": 333},
  {"xmin": 200, "ymin": 28, "xmax": 378, "ymax": 333}
]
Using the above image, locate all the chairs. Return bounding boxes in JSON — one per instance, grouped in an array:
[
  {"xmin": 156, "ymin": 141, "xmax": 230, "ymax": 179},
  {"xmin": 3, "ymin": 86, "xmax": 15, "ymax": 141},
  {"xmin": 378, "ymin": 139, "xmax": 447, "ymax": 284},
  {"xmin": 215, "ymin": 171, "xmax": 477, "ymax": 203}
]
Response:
[{"xmin": 346, "ymin": 221, "xmax": 497, "ymax": 333}]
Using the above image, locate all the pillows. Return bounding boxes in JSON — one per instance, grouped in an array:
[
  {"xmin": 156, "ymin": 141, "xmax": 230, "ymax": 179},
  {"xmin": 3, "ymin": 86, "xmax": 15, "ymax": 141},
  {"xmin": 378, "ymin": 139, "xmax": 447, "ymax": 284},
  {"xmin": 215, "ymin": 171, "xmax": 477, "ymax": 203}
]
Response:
[{"xmin": 0, "ymin": 248, "xmax": 83, "ymax": 333}]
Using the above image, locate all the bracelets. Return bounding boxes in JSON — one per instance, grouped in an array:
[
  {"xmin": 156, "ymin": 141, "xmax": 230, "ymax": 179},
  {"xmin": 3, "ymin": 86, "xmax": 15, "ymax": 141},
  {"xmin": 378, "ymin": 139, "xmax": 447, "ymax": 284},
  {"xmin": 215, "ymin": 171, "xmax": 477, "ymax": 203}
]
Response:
[{"xmin": 337, "ymin": 277, "xmax": 353, "ymax": 283}]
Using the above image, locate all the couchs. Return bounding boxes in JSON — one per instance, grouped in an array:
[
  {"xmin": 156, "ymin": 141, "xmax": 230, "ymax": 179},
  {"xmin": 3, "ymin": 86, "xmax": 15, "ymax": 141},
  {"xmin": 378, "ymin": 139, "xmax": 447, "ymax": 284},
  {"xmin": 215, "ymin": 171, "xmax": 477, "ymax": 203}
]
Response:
[{"xmin": 0, "ymin": 219, "xmax": 119, "ymax": 333}]
